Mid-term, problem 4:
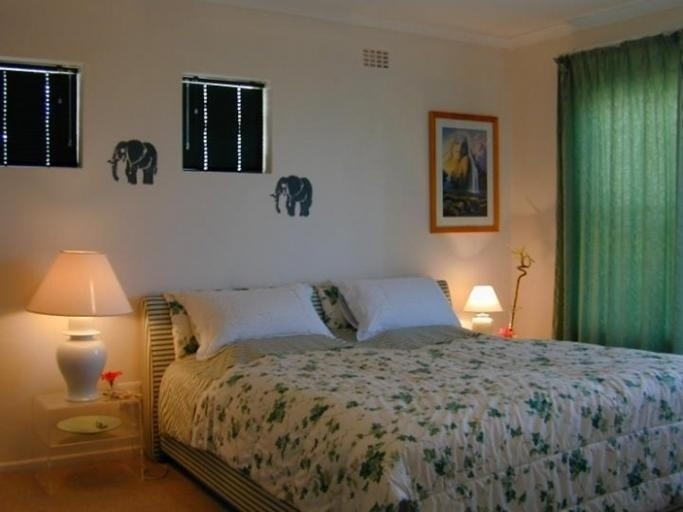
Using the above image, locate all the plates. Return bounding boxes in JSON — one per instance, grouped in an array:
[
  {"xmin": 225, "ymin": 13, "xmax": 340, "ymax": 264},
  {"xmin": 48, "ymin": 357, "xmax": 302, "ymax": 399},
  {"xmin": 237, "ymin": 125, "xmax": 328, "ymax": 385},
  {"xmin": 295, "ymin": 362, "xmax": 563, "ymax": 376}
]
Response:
[{"xmin": 56, "ymin": 414, "xmax": 123, "ymax": 435}]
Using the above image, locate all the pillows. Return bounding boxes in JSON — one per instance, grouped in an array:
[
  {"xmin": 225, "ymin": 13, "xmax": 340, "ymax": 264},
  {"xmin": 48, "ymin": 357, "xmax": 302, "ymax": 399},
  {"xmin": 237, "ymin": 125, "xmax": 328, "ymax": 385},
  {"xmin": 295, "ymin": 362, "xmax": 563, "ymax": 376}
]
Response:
[
  {"xmin": 173, "ymin": 284, "xmax": 336, "ymax": 363},
  {"xmin": 334, "ymin": 281, "xmax": 461, "ymax": 341},
  {"xmin": 165, "ymin": 286, "xmax": 286, "ymax": 362},
  {"xmin": 314, "ymin": 283, "xmax": 357, "ymax": 332}
]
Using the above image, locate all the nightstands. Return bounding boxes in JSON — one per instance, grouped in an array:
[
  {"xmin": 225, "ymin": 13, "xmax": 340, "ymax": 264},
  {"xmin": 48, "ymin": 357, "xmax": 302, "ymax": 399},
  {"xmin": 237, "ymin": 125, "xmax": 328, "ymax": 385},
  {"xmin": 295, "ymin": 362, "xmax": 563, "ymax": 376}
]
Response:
[{"xmin": 30, "ymin": 389, "xmax": 145, "ymax": 492}]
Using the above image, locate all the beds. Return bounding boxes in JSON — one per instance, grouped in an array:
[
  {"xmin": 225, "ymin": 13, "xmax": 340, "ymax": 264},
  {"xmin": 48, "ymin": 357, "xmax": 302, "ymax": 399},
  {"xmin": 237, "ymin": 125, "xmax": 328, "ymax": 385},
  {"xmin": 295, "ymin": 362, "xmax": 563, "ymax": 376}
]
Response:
[{"xmin": 138, "ymin": 279, "xmax": 683, "ymax": 512}]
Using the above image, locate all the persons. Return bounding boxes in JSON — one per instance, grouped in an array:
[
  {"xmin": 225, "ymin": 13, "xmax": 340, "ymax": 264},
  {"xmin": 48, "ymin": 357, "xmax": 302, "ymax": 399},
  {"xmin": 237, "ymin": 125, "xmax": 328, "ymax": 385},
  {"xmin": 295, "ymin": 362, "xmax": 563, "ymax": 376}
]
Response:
[{"xmin": 442, "ymin": 131, "xmax": 487, "ymax": 194}]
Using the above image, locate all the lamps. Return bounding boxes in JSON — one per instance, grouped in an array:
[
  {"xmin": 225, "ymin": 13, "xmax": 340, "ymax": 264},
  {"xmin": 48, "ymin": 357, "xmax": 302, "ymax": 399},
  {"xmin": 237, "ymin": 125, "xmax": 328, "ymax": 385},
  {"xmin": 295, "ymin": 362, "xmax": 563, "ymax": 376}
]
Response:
[
  {"xmin": 23, "ymin": 251, "xmax": 135, "ymax": 404},
  {"xmin": 463, "ymin": 282, "xmax": 504, "ymax": 333}
]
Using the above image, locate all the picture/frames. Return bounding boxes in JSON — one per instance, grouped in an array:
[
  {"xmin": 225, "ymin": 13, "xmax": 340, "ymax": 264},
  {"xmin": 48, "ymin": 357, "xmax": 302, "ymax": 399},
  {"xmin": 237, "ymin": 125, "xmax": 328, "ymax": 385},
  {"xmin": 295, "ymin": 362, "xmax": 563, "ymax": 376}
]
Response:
[{"xmin": 428, "ymin": 110, "xmax": 502, "ymax": 233}]
